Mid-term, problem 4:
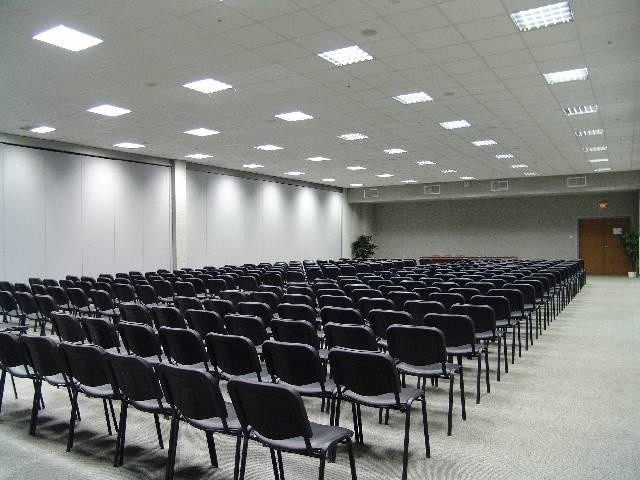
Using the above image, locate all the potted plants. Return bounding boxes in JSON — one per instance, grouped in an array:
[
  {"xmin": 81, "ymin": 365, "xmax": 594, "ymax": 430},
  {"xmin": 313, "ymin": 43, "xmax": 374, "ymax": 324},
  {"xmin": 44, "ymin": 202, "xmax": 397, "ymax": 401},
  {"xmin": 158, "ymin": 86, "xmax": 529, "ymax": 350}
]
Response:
[{"xmin": 619, "ymin": 228, "xmax": 638, "ymax": 279}]
